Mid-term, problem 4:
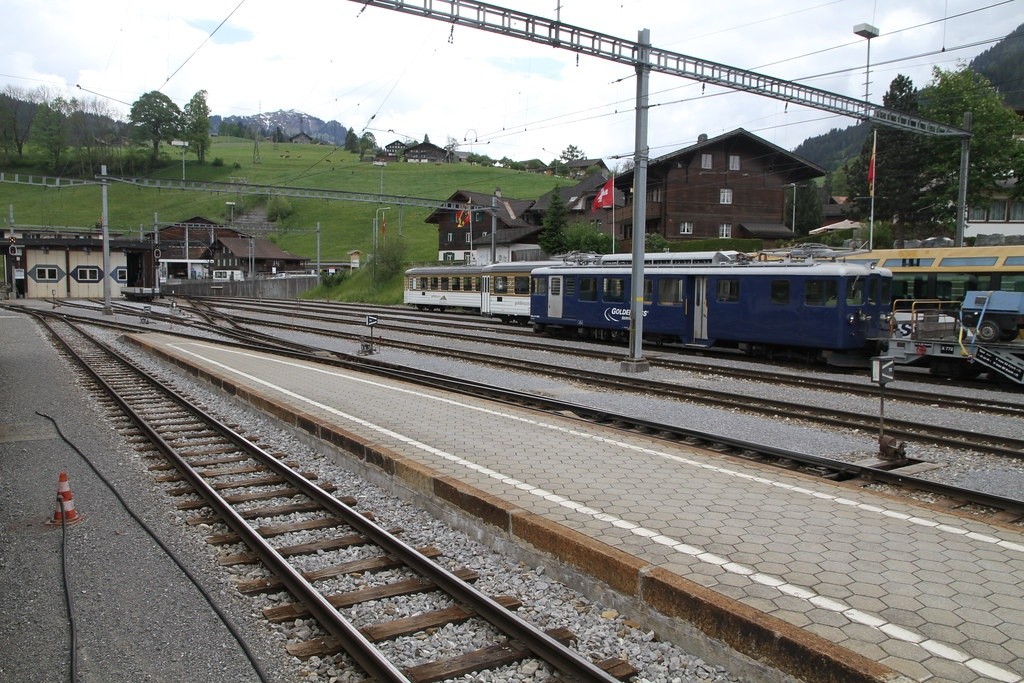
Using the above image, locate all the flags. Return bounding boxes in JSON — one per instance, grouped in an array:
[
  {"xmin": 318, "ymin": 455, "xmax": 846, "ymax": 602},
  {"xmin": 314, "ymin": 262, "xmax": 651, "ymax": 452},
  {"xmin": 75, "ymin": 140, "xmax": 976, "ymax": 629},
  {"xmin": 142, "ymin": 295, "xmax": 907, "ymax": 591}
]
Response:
[
  {"xmin": 590, "ymin": 175, "xmax": 614, "ymax": 213},
  {"xmin": 866, "ymin": 142, "xmax": 876, "ymax": 198},
  {"xmin": 456, "ymin": 202, "xmax": 471, "ymax": 228}
]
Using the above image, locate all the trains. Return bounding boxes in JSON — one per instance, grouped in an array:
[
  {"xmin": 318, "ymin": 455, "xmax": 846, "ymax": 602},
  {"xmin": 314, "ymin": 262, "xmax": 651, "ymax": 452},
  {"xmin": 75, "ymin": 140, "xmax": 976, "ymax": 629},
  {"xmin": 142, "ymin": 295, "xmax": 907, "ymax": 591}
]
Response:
[{"xmin": 402, "ymin": 245, "xmax": 1023, "ymax": 385}]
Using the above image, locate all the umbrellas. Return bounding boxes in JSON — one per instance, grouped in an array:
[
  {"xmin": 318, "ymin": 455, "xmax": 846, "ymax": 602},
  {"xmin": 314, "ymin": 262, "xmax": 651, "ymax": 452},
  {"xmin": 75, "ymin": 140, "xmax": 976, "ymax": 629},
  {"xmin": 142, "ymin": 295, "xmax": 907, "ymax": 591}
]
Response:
[{"xmin": 807, "ymin": 219, "xmax": 866, "ymax": 234}]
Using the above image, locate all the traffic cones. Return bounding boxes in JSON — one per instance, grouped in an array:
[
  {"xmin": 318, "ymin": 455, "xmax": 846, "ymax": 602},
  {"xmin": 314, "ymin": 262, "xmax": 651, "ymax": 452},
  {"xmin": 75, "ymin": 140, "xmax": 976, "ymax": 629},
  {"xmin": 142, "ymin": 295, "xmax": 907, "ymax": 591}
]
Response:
[{"xmin": 45, "ymin": 471, "xmax": 86, "ymax": 528}]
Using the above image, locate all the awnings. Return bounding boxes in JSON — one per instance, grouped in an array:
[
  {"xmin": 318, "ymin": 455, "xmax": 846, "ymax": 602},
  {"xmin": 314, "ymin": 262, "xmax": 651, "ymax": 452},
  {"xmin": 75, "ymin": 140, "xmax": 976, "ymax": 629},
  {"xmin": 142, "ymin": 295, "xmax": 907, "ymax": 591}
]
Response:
[{"xmin": 740, "ymin": 222, "xmax": 798, "ymax": 240}]
